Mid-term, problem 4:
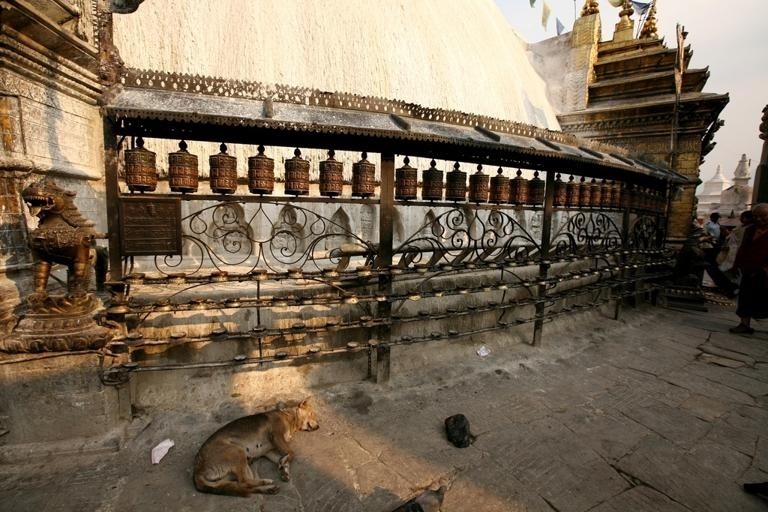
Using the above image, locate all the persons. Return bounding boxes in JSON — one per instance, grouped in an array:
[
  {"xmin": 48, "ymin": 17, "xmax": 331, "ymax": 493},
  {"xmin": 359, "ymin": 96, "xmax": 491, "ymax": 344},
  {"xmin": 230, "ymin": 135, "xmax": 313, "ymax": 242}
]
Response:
[{"xmin": 701, "ymin": 203, "xmax": 768, "ymax": 334}]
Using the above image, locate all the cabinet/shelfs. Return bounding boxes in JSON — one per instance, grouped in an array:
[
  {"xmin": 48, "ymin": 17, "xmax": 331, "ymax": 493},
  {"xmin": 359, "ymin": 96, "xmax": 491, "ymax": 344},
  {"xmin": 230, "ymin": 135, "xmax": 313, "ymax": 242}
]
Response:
[{"xmin": 102, "ymin": 244, "xmax": 675, "ymax": 373}]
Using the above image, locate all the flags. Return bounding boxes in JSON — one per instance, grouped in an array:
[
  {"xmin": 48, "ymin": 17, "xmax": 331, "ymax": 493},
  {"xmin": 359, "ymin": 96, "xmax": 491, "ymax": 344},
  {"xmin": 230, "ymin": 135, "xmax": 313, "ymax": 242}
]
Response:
[
  {"xmin": 528, "ymin": 1, "xmax": 565, "ymax": 37},
  {"xmin": 628, "ymin": 0, "xmax": 651, "ymax": 18}
]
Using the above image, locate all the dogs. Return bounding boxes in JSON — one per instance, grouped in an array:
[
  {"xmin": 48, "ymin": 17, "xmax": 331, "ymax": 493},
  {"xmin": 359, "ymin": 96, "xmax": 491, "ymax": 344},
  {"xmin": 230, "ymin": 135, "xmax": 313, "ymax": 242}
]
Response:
[
  {"xmin": 392, "ymin": 486, "xmax": 447, "ymax": 512},
  {"xmin": 192, "ymin": 395, "xmax": 320, "ymax": 497}
]
